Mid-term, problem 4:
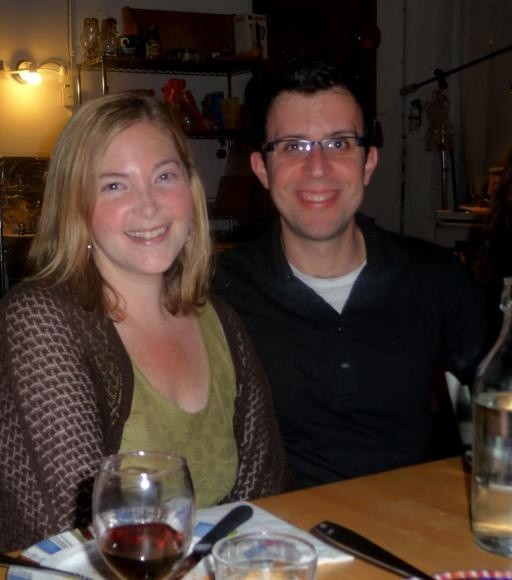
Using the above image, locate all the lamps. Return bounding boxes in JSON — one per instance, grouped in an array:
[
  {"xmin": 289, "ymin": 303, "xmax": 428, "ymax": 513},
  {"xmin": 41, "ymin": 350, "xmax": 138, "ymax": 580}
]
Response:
[{"xmin": 14, "ymin": 61, "xmax": 68, "ymax": 87}]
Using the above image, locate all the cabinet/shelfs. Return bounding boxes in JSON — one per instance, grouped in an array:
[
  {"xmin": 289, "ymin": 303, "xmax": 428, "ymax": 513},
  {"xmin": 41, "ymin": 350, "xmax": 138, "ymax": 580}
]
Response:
[{"xmin": 76, "ymin": 49, "xmax": 261, "ymax": 253}]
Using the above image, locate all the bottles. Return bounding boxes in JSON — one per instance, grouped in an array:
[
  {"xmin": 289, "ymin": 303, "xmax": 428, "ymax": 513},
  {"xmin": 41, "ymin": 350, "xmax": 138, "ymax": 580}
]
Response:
[
  {"xmin": 81, "ymin": 18, "xmax": 119, "ymax": 60},
  {"xmin": 470, "ymin": 278, "xmax": 512, "ymax": 558}
]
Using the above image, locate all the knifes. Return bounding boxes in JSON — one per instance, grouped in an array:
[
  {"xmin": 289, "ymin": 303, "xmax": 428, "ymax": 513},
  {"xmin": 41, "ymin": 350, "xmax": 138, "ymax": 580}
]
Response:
[{"xmin": 165, "ymin": 504, "xmax": 253, "ymax": 580}]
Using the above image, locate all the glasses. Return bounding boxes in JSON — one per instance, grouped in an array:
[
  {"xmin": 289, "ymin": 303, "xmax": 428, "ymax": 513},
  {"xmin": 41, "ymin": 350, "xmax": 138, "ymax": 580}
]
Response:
[{"xmin": 262, "ymin": 134, "xmax": 367, "ymax": 161}]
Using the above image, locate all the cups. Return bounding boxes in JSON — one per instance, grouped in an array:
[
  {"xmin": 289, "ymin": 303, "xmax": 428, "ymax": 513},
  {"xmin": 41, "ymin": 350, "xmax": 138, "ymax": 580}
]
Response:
[
  {"xmin": 91, "ymin": 449, "xmax": 194, "ymax": 579},
  {"xmin": 208, "ymin": 532, "xmax": 318, "ymax": 580}
]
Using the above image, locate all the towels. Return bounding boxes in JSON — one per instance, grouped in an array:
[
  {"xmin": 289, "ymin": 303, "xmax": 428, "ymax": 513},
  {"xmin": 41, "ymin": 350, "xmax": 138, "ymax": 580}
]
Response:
[{"xmin": 193, "ymin": 501, "xmax": 354, "ymax": 566}]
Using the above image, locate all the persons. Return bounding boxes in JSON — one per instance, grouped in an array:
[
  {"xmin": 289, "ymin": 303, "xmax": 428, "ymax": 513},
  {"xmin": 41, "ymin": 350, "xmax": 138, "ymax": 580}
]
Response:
[
  {"xmin": 183, "ymin": 43, "xmax": 508, "ymax": 495},
  {"xmin": 2, "ymin": 88, "xmax": 289, "ymax": 558}
]
